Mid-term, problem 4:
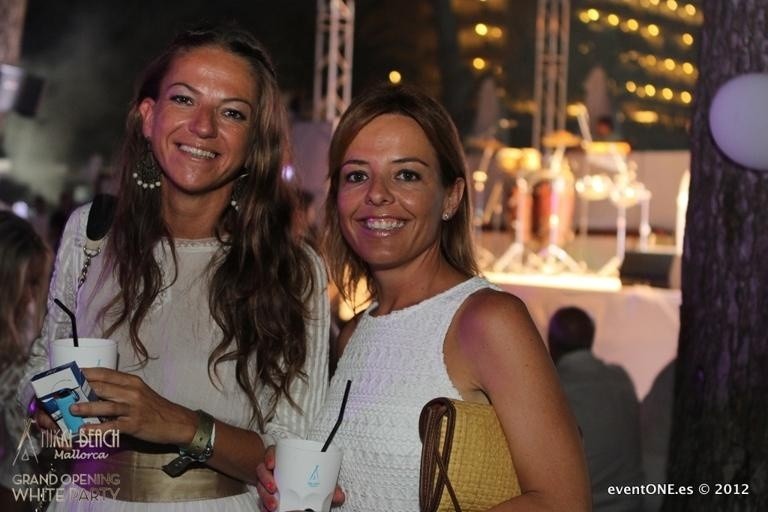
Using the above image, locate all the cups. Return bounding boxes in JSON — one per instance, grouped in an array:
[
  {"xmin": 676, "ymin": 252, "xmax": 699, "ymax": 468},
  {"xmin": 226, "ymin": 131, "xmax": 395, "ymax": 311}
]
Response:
[
  {"xmin": 271, "ymin": 438, "xmax": 344, "ymax": 512},
  {"xmin": 48, "ymin": 337, "xmax": 117, "ymax": 446}
]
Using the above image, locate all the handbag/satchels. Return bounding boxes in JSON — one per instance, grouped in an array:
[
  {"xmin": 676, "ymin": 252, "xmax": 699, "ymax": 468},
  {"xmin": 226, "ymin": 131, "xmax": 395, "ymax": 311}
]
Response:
[{"xmin": 417, "ymin": 396, "xmax": 585, "ymax": 512}]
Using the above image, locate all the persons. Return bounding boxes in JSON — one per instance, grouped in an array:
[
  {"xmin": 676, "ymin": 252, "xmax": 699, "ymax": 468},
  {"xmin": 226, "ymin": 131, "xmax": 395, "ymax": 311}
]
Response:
[
  {"xmin": 257, "ymin": 87, "xmax": 594, "ymax": 512},
  {"xmin": 49, "ymin": 190, "xmax": 76, "ymax": 254},
  {"xmin": 1, "ymin": 209, "xmax": 53, "ymax": 511},
  {"xmin": 15, "ymin": 31, "xmax": 329, "ymax": 512},
  {"xmin": 286, "ymin": 188, "xmax": 319, "ymax": 257},
  {"xmin": 593, "ymin": 116, "xmax": 624, "ymax": 141},
  {"xmin": 548, "ymin": 308, "xmax": 647, "ymax": 512},
  {"xmin": 29, "ymin": 194, "xmax": 53, "ymax": 254}
]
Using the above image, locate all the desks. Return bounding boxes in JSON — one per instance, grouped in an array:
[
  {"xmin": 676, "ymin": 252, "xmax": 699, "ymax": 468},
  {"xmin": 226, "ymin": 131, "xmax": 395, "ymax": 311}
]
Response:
[{"xmin": 482, "ymin": 275, "xmax": 682, "ymax": 394}]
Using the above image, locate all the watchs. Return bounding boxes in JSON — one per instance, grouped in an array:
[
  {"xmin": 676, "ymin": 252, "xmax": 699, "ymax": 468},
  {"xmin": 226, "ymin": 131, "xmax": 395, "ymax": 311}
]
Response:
[{"xmin": 163, "ymin": 409, "xmax": 216, "ymax": 478}]
